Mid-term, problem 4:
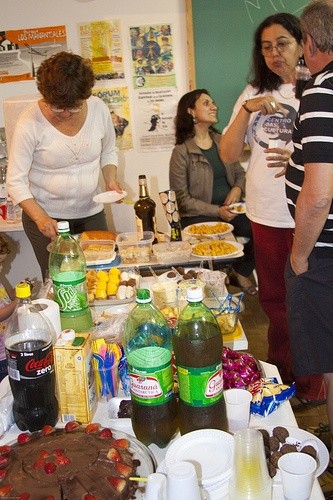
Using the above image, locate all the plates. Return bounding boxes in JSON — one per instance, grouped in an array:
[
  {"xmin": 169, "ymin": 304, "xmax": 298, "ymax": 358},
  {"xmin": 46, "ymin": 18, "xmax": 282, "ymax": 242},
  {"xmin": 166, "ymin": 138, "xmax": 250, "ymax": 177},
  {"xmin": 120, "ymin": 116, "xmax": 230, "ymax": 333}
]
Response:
[
  {"xmin": 253, "ymin": 426, "xmax": 330, "ymax": 486},
  {"xmin": 164, "ymin": 429, "xmax": 234, "ymax": 494},
  {"xmin": 190, "ymin": 240, "xmax": 244, "ymax": 258},
  {"xmin": 46, "ymin": 234, "xmax": 80, "ymax": 256},
  {"xmin": 92, "ymin": 190, "xmax": 127, "ymax": 203},
  {"xmin": 184, "ymin": 222, "xmax": 234, "ymax": 235},
  {"xmin": 229, "ymin": 203, "xmax": 245, "ymax": 214},
  {"xmin": 158, "ymin": 268, "xmax": 211, "ymax": 283}
]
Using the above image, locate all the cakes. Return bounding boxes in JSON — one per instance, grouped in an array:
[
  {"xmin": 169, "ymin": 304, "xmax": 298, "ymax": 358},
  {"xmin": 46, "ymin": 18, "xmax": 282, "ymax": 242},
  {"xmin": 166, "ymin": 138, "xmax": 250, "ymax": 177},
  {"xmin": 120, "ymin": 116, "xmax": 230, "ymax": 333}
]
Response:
[{"xmin": 0, "ymin": 420, "xmax": 140, "ymax": 500}]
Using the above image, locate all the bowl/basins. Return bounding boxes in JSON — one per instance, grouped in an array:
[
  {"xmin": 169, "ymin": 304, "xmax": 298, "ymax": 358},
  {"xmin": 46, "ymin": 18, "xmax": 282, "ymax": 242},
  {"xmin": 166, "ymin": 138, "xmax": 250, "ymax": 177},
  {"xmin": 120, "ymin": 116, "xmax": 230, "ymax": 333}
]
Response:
[
  {"xmin": 116, "ymin": 231, "xmax": 155, "ymax": 264},
  {"xmin": 80, "ymin": 240, "xmax": 115, "ymax": 260},
  {"xmin": 153, "ymin": 241, "xmax": 192, "ymax": 264}
]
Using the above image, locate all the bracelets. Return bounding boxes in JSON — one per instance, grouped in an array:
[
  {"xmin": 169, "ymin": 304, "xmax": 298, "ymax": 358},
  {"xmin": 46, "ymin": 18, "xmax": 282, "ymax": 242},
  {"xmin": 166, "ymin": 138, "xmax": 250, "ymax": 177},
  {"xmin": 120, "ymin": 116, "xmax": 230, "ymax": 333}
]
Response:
[{"xmin": 241, "ymin": 100, "xmax": 252, "ymax": 113}]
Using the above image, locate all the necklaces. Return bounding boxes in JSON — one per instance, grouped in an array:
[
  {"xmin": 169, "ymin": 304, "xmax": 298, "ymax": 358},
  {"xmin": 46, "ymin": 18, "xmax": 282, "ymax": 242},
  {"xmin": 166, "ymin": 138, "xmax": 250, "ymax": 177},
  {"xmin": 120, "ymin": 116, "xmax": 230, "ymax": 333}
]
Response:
[{"xmin": 278, "ymin": 88, "xmax": 295, "ymax": 100}]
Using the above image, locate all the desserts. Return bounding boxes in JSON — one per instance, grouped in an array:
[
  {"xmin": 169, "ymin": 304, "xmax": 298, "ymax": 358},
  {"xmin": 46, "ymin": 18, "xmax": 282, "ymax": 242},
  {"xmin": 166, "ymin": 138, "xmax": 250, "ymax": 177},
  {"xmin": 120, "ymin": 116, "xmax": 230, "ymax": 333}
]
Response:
[
  {"xmin": 166, "ymin": 266, "xmax": 202, "ymax": 285},
  {"xmin": 84, "ymin": 267, "xmax": 138, "ymax": 303},
  {"xmin": 256, "ymin": 426, "xmax": 317, "ymax": 477}
]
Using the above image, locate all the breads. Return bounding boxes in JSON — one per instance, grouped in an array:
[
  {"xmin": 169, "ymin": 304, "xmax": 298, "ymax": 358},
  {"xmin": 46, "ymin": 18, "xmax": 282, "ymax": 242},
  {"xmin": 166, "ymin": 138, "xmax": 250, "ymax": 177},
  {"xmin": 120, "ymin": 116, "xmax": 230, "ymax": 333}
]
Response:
[{"xmin": 58, "ymin": 230, "xmax": 122, "ymax": 253}]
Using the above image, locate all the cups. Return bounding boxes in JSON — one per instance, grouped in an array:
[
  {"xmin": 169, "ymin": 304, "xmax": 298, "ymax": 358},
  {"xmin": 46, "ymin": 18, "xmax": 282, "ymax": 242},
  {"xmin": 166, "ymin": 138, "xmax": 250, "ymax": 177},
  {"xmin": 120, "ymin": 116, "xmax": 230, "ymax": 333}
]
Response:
[
  {"xmin": 199, "ymin": 271, "xmax": 227, "ymax": 297},
  {"xmin": 144, "ymin": 473, "xmax": 167, "ymax": 500},
  {"xmin": 166, "ymin": 462, "xmax": 200, "ymax": 500},
  {"xmin": 223, "ymin": 389, "xmax": 253, "ymax": 434},
  {"xmin": 93, "ymin": 360, "xmax": 120, "ymax": 402},
  {"xmin": 278, "ymin": 452, "xmax": 317, "ymax": 500},
  {"xmin": 269, "ymin": 140, "xmax": 287, "ymax": 162},
  {"xmin": 230, "ymin": 429, "xmax": 274, "ymax": 500}
]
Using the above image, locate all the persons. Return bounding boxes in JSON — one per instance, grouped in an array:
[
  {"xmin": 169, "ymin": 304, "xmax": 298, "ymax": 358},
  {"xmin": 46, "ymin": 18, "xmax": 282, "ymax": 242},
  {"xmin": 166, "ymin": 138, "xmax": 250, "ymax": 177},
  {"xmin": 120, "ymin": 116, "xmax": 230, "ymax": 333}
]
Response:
[
  {"xmin": 168, "ymin": 89, "xmax": 259, "ymax": 295},
  {"xmin": 264, "ymin": 0, "xmax": 333, "ymax": 473},
  {"xmin": 220, "ymin": 13, "xmax": 321, "ymax": 229},
  {"xmin": 5, "ymin": 51, "xmax": 122, "ymax": 286}
]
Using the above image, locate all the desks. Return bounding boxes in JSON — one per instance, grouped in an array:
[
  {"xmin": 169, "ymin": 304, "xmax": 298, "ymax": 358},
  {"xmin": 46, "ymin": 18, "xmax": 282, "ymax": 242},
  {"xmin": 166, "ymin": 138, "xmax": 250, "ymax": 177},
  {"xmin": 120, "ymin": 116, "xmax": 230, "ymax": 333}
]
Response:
[{"xmin": 0, "ymin": 231, "xmax": 327, "ymax": 500}]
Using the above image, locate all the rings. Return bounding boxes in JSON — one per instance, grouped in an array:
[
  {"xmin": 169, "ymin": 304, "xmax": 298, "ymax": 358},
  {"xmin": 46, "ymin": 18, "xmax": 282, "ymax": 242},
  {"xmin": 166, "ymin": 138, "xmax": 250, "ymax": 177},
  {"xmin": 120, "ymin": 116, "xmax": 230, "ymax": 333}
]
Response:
[{"xmin": 270, "ymin": 102, "xmax": 275, "ymax": 109}]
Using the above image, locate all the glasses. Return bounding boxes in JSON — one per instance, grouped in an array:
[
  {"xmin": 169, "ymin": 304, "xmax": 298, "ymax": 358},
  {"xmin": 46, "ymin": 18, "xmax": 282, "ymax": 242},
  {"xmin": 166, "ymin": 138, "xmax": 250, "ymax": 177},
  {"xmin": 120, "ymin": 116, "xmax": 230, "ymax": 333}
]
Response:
[
  {"xmin": 260, "ymin": 39, "xmax": 299, "ymax": 57},
  {"xmin": 48, "ymin": 103, "xmax": 85, "ymax": 114}
]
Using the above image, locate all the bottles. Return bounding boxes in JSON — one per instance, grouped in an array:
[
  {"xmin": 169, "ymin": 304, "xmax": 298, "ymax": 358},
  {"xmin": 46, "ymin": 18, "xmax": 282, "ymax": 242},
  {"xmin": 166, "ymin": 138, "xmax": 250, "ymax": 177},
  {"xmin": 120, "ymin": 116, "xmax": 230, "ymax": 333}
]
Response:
[
  {"xmin": 134, "ymin": 175, "xmax": 158, "ymax": 245},
  {"xmin": 6, "ymin": 197, "xmax": 18, "ymax": 225},
  {"xmin": 294, "ymin": 59, "xmax": 309, "ymax": 99},
  {"xmin": 122, "ymin": 289, "xmax": 178, "ymax": 449},
  {"xmin": 48, "ymin": 221, "xmax": 93, "ymax": 333},
  {"xmin": 174, "ymin": 287, "xmax": 228, "ymax": 437},
  {"xmin": 4, "ymin": 283, "xmax": 59, "ymax": 432}
]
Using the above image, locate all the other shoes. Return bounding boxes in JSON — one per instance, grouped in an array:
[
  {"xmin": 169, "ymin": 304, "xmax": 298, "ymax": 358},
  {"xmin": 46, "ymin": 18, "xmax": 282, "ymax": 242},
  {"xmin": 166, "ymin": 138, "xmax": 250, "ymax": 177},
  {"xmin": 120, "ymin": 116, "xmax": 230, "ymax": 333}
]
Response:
[
  {"xmin": 288, "ymin": 394, "xmax": 328, "ymax": 412},
  {"xmin": 230, "ymin": 275, "xmax": 258, "ymax": 297}
]
